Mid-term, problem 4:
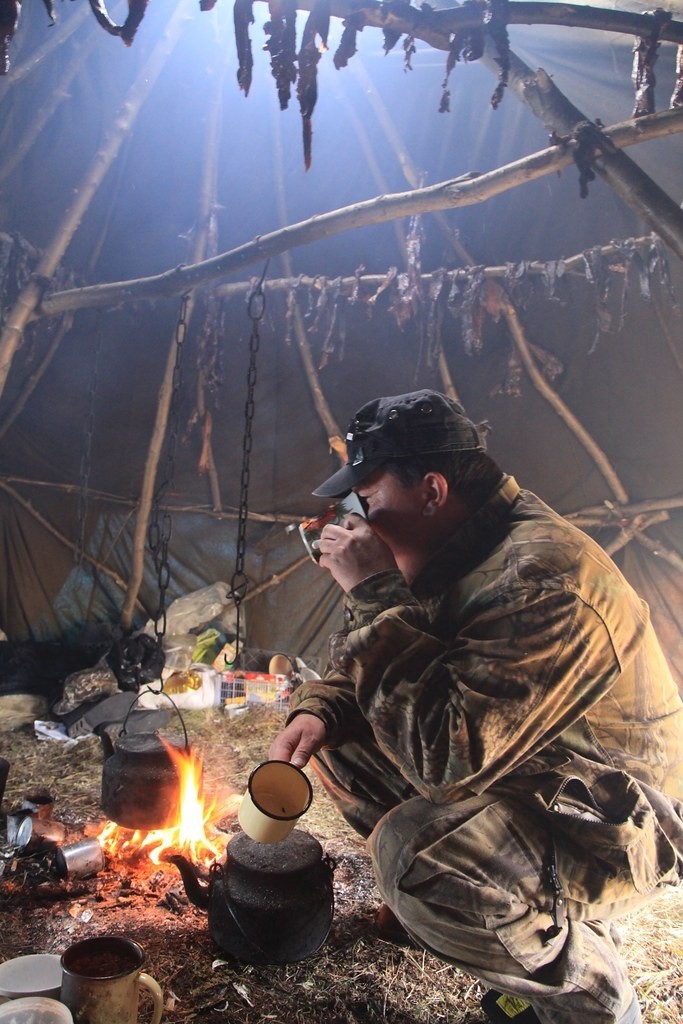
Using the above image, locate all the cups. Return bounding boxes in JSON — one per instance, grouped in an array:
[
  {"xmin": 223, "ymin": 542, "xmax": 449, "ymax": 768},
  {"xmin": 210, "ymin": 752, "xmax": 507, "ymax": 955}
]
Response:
[
  {"xmin": 14, "ymin": 816, "xmax": 70, "ymax": 850},
  {"xmin": 7, "ymin": 809, "xmax": 38, "ymax": 845},
  {"xmin": 235, "ymin": 760, "xmax": 314, "ymax": 843},
  {"xmin": 299, "ymin": 491, "xmax": 369, "ymax": 565},
  {"xmin": 22, "ymin": 795, "xmax": 55, "ymax": 820},
  {"xmin": 59, "ymin": 937, "xmax": 164, "ymax": 1024},
  {"xmin": 57, "ymin": 838, "xmax": 106, "ymax": 881},
  {"xmin": 1, "ymin": 996, "xmax": 73, "ymax": 1024}
]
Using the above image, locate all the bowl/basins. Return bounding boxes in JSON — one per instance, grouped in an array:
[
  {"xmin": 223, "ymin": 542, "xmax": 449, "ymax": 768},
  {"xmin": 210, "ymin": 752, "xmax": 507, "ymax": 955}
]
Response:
[{"xmin": 0, "ymin": 953, "xmax": 65, "ymax": 1000}]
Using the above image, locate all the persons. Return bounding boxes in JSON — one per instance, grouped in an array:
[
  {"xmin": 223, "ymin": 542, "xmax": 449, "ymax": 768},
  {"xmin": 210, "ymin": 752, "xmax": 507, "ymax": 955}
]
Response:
[{"xmin": 266, "ymin": 389, "xmax": 681, "ymax": 1021}]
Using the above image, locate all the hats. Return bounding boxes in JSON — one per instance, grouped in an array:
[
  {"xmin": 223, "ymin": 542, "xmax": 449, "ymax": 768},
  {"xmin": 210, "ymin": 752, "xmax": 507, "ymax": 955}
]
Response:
[{"xmin": 311, "ymin": 387, "xmax": 488, "ymax": 499}]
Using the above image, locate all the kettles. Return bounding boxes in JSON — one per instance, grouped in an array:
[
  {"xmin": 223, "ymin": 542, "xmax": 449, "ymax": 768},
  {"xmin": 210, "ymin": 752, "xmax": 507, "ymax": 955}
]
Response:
[
  {"xmin": 97, "ymin": 689, "xmax": 205, "ymax": 832},
  {"xmin": 168, "ymin": 828, "xmax": 339, "ymax": 965}
]
